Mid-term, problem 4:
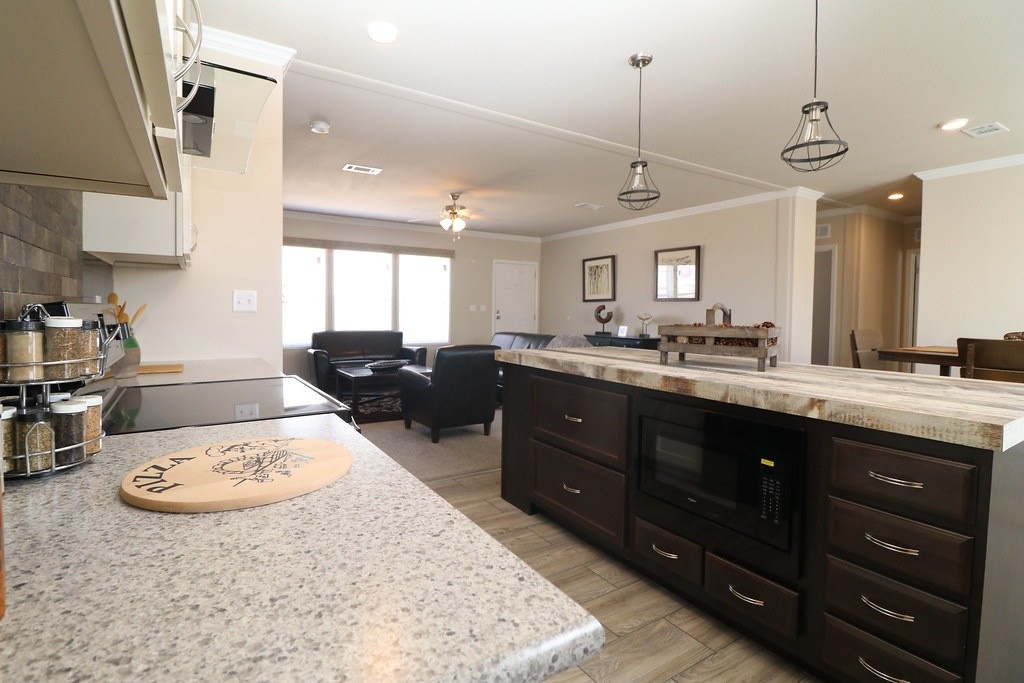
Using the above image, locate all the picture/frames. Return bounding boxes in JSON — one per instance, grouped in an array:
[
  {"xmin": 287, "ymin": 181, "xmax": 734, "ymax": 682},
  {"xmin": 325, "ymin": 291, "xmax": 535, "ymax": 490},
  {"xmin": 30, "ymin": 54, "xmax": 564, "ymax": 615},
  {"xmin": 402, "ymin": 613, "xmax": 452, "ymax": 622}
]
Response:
[
  {"xmin": 582, "ymin": 255, "xmax": 616, "ymax": 302},
  {"xmin": 654, "ymin": 245, "xmax": 700, "ymax": 301}
]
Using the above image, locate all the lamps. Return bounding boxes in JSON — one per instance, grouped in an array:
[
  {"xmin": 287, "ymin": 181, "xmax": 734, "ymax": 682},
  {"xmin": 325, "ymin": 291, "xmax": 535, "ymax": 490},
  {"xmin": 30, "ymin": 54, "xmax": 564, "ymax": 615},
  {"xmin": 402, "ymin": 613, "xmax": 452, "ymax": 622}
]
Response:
[
  {"xmin": 441, "ymin": 212, "xmax": 467, "ymax": 241},
  {"xmin": 618, "ymin": 51, "xmax": 660, "ymax": 211},
  {"xmin": 780, "ymin": 0, "xmax": 849, "ymax": 173}
]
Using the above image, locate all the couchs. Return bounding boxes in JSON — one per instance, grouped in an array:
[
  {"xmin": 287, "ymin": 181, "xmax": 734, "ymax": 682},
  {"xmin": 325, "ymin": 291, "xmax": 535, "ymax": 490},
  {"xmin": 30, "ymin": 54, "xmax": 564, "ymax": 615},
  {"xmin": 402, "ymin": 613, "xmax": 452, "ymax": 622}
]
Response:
[
  {"xmin": 307, "ymin": 331, "xmax": 427, "ymax": 393},
  {"xmin": 490, "ymin": 332, "xmax": 592, "ymax": 407},
  {"xmin": 397, "ymin": 344, "xmax": 501, "ymax": 443}
]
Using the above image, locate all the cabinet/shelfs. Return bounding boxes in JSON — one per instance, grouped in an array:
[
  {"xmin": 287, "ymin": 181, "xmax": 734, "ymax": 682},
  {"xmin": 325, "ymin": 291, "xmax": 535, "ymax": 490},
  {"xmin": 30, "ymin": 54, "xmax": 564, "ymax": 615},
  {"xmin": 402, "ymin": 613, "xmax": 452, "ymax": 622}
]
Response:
[
  {"xmin": 499, "ymin": 361, "xmax": 1024, "ymax": 683},
  {"xmin": 0, "ymin": 0, "xmax": 199, "ymax": 270}
]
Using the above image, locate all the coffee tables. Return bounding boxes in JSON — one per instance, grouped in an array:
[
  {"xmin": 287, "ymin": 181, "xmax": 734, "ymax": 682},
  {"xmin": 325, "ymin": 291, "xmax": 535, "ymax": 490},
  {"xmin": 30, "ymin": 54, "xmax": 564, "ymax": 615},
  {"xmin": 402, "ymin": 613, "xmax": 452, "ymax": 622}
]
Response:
[{"xmin": 336, "ymin": 368, "xmax": 432, "ymax": 413}]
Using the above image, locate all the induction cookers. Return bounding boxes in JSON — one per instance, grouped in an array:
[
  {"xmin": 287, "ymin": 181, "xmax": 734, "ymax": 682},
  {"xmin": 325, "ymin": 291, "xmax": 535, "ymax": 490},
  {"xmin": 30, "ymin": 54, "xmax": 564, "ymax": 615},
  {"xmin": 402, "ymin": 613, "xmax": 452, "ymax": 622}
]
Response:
[{"xmin": 40, "ymin": 298, "xmax": 353, "ymax": 436}]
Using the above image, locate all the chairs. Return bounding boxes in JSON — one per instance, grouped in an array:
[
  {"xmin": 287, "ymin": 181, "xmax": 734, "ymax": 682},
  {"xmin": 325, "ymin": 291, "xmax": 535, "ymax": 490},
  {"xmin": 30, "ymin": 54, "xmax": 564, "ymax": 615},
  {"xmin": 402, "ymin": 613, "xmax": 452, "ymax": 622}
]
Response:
[
  {"xmin": 957, "ymin": 338, "xmax": 1024, "ymax": 383},
  {"xmin": 850, "ymin": 330, "xmax": 887, "ymax": 370}
]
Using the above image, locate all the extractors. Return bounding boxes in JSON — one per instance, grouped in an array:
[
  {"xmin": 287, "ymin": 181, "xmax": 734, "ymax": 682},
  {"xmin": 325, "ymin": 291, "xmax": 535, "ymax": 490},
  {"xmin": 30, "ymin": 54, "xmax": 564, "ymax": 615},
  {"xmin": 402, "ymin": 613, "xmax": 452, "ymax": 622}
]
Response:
[{"xmin": 182, "ymin": 56, "xmax": 277, "ymax": 174}]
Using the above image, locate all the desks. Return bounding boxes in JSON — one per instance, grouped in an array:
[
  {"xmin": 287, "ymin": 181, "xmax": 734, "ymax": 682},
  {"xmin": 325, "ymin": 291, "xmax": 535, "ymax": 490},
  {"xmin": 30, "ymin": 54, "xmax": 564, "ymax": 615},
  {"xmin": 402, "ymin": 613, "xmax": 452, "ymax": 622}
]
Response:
[
  {"xmin": 583, "ymin": 335, "xmax": 661, "ymax": 350},
  {"xmin": 876, "ymin": 345, "xmax": 960, "ymax": 377}
]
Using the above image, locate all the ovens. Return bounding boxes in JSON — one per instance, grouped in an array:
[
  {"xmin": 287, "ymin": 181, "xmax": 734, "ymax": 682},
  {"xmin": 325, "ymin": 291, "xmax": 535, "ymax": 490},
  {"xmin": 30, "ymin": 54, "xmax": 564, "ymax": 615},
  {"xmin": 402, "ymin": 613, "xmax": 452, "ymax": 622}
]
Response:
[{"xmin": 625, "ymin": 393, "xmax": 809, "ymax": 583}]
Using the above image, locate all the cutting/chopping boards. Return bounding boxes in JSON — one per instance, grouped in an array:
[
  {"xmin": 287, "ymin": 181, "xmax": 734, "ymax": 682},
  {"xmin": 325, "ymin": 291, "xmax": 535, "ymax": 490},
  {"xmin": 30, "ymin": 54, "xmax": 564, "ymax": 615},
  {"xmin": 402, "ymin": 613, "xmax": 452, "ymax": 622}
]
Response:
[{"xmin": 119, "ymin": 437, "xmax": 353, "ymax": 513}]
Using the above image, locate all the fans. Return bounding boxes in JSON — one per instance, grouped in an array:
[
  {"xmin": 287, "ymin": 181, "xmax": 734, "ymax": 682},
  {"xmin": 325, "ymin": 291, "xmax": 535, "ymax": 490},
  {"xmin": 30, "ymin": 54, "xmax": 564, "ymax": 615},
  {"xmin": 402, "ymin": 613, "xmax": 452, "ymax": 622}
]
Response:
[{"xmin": 408, "ymin": 192, "xmax": 491, "ymax": 221}]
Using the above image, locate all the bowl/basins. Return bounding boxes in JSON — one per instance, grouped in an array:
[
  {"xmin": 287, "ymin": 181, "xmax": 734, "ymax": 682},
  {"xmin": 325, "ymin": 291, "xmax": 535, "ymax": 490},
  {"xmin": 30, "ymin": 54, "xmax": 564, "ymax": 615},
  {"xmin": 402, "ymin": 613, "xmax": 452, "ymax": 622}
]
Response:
[{"xmin": 365, "ymin": 359, "xmax": 408, "ymax": 372}]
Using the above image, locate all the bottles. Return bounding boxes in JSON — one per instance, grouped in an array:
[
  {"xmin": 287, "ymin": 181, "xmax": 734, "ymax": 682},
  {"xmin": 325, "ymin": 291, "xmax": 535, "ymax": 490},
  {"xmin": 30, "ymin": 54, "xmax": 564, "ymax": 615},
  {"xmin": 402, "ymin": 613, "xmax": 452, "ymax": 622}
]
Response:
[{"xmin": 0, "ymin": 313, "xmax": 102, "ymax": 471}]
